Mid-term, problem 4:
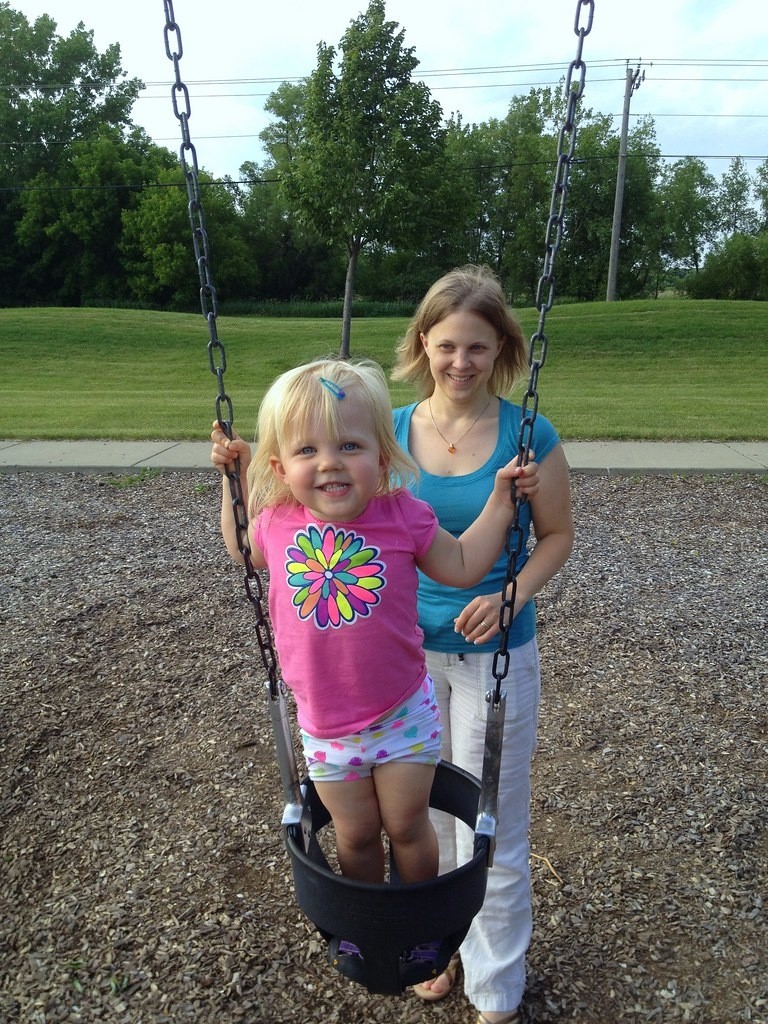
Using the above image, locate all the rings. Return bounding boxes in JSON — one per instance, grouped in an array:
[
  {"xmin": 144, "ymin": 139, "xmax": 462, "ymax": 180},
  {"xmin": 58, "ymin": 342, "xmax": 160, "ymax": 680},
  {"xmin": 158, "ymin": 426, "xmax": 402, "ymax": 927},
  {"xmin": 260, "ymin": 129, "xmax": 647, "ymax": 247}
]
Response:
[{"xmin": 481, "ymin": 621, "xmax": 489, "ymax": 630}]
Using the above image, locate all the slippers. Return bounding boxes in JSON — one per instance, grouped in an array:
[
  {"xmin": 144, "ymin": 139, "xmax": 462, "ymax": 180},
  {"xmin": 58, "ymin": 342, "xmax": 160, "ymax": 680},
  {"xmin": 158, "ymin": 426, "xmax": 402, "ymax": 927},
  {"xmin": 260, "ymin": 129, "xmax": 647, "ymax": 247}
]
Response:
[
  {"xmin": 413, "ymin": 953, "xmax": 461, "ymax": 1000},
  {"xmin": 476, "ymin": 1010, "xmax": 521, "ymax": 1024}
]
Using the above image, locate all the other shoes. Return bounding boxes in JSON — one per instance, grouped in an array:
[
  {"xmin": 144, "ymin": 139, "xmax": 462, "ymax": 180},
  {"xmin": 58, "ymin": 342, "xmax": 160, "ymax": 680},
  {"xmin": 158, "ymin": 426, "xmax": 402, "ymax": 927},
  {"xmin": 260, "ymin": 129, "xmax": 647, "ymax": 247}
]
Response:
[
  {"xmin": 339, "ymin": 941, "xmax": 362, "ymax": 960},
  {"xmin": 408, "ymin": 941, "xmax": 439, "ymax": 967}
]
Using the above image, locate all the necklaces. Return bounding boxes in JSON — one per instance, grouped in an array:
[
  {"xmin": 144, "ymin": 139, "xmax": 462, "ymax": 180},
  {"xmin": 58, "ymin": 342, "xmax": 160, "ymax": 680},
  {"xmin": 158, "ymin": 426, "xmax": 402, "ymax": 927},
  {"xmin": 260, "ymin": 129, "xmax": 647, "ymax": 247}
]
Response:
[{"xmin": 431, "ymin": 398, "xmax": 492, "ymax": 454}]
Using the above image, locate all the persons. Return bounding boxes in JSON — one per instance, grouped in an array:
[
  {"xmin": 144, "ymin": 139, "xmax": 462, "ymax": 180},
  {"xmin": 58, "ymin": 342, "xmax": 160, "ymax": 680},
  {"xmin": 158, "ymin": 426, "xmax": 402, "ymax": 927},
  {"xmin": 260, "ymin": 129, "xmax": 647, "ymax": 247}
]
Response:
[
  {"xmin": 209, "ymin": 360, "xmax": 540, "ymax": 885},
  {"xmin": 380, "ymin": 266, "xmax": 575, "ymax": 1024}
]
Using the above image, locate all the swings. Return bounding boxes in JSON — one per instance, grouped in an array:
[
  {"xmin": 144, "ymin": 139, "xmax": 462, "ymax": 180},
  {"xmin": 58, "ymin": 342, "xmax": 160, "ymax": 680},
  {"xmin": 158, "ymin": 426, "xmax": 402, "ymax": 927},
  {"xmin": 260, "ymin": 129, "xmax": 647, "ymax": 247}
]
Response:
[{"xmin": 154, "ymin": 1, "xmax": 596, "ymax": 1000}]
